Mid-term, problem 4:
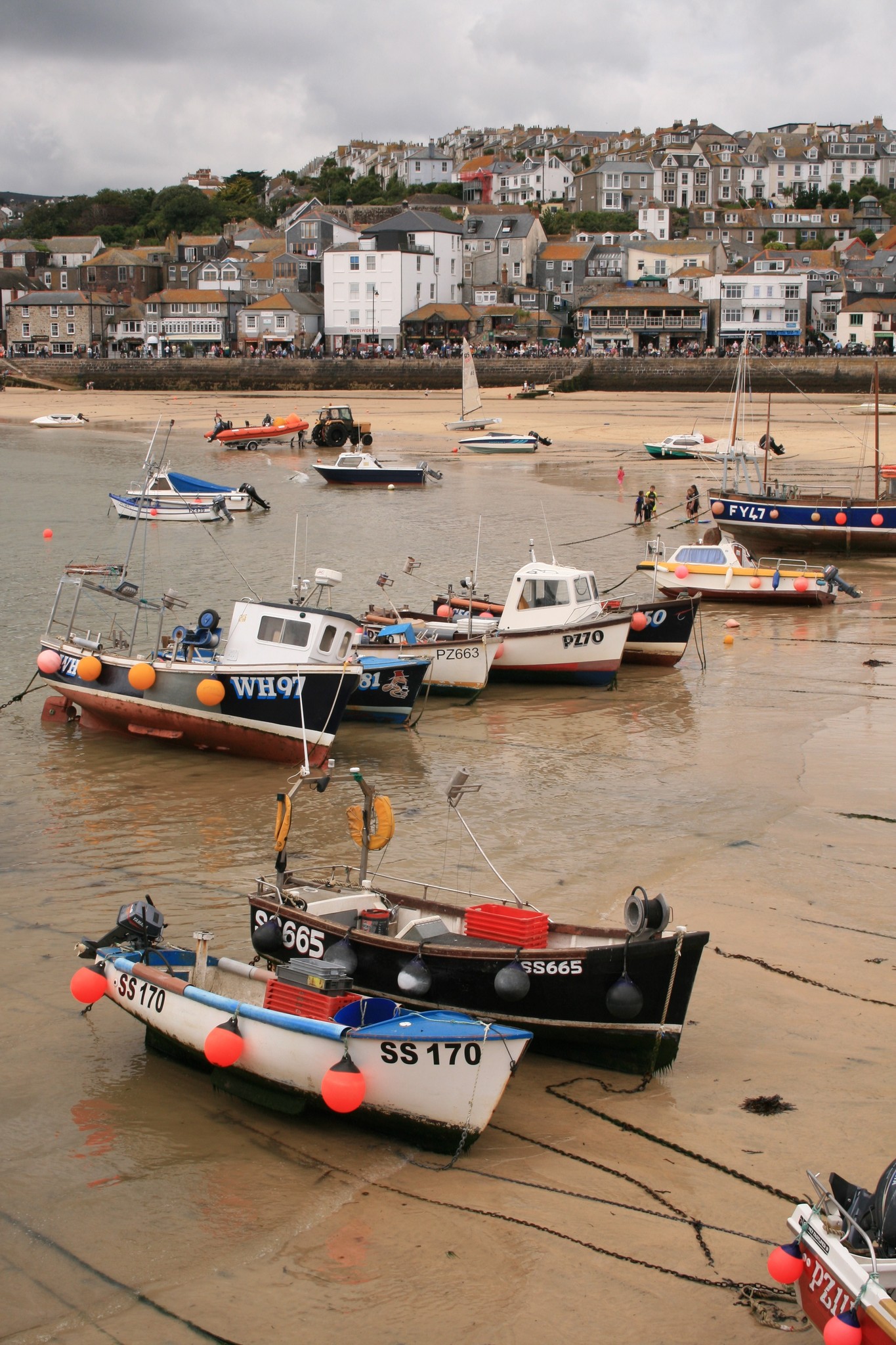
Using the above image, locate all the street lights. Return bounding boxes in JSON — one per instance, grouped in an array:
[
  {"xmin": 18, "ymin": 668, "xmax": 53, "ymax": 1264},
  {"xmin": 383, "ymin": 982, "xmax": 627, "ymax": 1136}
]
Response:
[
  {"xmin": 228, "ymin": 286, "xmax": 236, "ymax": 357},
  {"xmin": 718, "ymin": 279, "xmax": 726, "ymax": 357},
  {"xmin": 372, "ymin": 286, "xmax": 380, "ymax": 359},
  {"xmin": 85, "ymin": 292, "xmax": 93, "ymax": 359},
  {"xmin": 537, "ymin": 284, "xmax": 544, "ymax": 358}
]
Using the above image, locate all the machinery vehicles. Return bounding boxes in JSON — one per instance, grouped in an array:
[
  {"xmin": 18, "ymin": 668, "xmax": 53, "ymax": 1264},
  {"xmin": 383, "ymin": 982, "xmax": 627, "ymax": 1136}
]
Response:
[{"xmin": 311, "ymin": 403, "xmax": 373, "ymax": 447}]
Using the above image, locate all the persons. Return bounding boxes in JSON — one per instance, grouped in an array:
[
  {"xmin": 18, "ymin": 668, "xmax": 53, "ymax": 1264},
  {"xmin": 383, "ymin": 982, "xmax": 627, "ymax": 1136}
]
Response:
[
  {"xmin": 633, "ymin": 485, "xmax": 702, "ymax": 525},
  {"xmin": 86, "ymin": 382, "xmax": 94, "ymax": 390},
  {"xmin": 617, "ymin": 466, "xmax": 624, "ymax": 492},
  {"xmin": 3, "ymin": 368, "xmax": 8, "ymax": 380},
  {"xmin": 0, "ymin": 325, "xmax": 889, "ymax": 358}
]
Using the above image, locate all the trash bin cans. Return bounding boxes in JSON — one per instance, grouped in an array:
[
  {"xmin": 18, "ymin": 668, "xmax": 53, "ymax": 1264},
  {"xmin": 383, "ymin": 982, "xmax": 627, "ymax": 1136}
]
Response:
[
  {"xmin": 622, "ymin": 346, "xmax": 633, "ymax": 358},
  {"xmin": 804, "ymin": 346, "xmax": 815, "ymax": 357},
  {"xmin": 451, "ymin": 347, "xmax": 460, "ymax": 358},
  {"xmin": 9, "ymin": 350, "xmax": 14, "ymax": 358},
  {"xmin": 311, "ymin": 351, "xmax": 316, "ymax": 359},
  {"xmin": 716, "ymin": 347, "xmax": 723, "ymax": 357},
  {"xmin": 225, "ymin": 348, "xmax": 230, "ymax": 355},
  {"xmin": 299, "ymin": 348, "xmax": 309, "ymax": 359}
]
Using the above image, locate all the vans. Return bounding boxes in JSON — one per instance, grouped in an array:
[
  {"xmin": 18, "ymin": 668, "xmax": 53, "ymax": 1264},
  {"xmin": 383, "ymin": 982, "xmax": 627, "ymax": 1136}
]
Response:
[{"xmin": 357, "ymin": 342, "xmax": 390, "ymax": 359}]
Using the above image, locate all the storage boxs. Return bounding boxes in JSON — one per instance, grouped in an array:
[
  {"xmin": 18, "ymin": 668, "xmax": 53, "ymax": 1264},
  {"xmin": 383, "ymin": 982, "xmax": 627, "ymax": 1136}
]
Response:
[
  {"xmin": 424, "ymin": 618, "xmax": 498, "ymax": 633},
  {"xmin": 464, "ymin": 903, "xmax": 550, "ymax": 949},
  {"xmin": 263, "ymin": 958, "xmax": 362, "ymax": 1023}
]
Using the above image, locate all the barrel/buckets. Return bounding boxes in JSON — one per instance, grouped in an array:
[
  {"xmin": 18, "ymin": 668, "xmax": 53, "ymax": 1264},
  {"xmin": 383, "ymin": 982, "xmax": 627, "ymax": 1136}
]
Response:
[{"xmin": 361, "ymin": 909, "xmax": 390, "ymax": 936}]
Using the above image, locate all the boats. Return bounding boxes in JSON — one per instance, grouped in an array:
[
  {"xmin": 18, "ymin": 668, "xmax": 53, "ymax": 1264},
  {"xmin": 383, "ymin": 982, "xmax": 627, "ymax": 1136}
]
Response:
[
  {"xmin": 457, "ymin": 430, "xmax": 553, "ymax": 454},
  {"xmin": 311, "ymin": 420, "xmax": 444, "ymax": 486},
  {"xmin": 152, "ymin": 511, "xmax": 435, "ymax": 726},
  {"xmin": 351, "ymin": 504, "xmax": 705, "ymax": 699},
  {"xmin": 636, "ymin": 531, "xmax": 863, "ymax": 606},
  {"xmin": 785, "ymin": 1157, "xmax": 896, "ymax": 1345},
  {"xmin": 37, "ymin": 418, "xmax": 363, "ymax": 777},
  {"xmin": 108, "ymin": 492, "xmax": 233, "ymax": 522},
  {"xmin": 125, "ymin": 460, "xmax": 272, "ymax": 512},
  {"xmin": 841, "ymin": 402, "xmax": 896, "ymax": 415},
  {"xmin": 203, "ymin": 410, "xmax": 309, "ymax": 447},
  {"xmin": 642, "ymin": 418, "xmax": 718, "ymax": 459},
  {"xmin": 243, "ymin": 666, "xmax": 710, "ymax": 1078},
  {"xmin": 72, "ymin": 893, "xmax": 536, "ymax": 1157},
  {"xmin": 29, "ymin": 413, "xmax": 90, "ymax": 427},
  {"xmin": 684, "ymin": 434, "xmax": 785, "ymax": 462}
]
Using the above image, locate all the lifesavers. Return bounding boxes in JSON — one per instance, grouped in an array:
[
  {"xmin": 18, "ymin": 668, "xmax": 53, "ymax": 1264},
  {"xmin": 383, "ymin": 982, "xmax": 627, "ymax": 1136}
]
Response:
[
  {"xmin": 346, "ymin": 796, "xmax": 395, "ymax": 850},
  {"xmin": 275, "ymin": 792, "xmax": 292, "ymax": 852}
]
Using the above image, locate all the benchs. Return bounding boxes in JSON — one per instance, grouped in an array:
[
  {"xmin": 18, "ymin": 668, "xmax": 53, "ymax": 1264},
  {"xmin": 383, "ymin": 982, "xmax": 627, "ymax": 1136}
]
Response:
[
  {"xmin": 805, "ymin": 346, "xmax": 815, "ymax": 358},
  {"xmin": 332, "ymin": 351, "xmax": 357, "ymax": 359},
  {"xmin": 300, "ymin": 349, "xmax": 318, "ymax": 359},
  {"xmin": 839, "ymin": 348, "xmax": 852, "ymax": 358},
  {"xmin": 471, "ymin": 349, "xmax": 579, "ymax": 358},
  {"xmin": 251, "ymin": 351, "xmax": 262, "ymax": 359}
]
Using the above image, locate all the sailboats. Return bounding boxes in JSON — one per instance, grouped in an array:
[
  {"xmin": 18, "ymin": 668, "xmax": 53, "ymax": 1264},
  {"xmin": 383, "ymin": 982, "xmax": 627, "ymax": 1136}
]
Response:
[
  {"xmin": 441, "ymin": 336, "xmax": 502, "ymax": 431},
  {"xmin": 661, "ymin": 328, "xmax": 896, "ymax": 554}
]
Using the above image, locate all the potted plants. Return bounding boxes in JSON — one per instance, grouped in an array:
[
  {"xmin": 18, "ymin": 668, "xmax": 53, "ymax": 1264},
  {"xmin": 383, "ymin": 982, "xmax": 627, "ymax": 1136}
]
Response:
[
  {"xmin": 416, "ymin": 345, "xmax": 424, "ymax": 359},
  {"xmin": 183, "ymin": 344, "xmax": 195, "ymax": 358},
  {"xmin": 634, "ymin": 352, "xmax": 637, "ymax": 358}
]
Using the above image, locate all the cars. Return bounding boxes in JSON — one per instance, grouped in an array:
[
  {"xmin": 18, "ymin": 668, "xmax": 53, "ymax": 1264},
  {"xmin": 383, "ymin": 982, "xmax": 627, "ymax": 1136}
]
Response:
[{"xmin": 845, "ymin": 342, "xmax": 869, "ymax": 355}]
